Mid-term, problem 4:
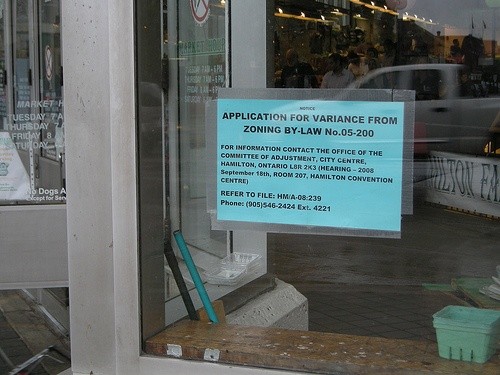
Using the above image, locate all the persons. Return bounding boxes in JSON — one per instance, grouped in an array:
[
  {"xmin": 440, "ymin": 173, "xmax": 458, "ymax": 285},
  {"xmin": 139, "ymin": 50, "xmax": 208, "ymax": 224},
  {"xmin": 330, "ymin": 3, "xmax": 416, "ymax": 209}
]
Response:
[
  {"xmin": 340, "ymin": 34, "xmax": 485, "ymax": 89},
  {"xmin": 278, "ymin": 49, "xmax": 318, "ymax": 89},
  {"xmin": 320, "ymin": 52, "xmax": 355, "ymax": 88}
]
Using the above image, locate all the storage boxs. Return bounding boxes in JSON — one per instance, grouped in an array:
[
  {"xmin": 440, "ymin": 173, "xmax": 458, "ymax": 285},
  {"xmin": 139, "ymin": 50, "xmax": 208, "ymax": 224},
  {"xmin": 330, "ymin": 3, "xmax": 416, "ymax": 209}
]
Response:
[{"xmin": 432, "ymin": 306, "xmax": 500, "ymax": 365}]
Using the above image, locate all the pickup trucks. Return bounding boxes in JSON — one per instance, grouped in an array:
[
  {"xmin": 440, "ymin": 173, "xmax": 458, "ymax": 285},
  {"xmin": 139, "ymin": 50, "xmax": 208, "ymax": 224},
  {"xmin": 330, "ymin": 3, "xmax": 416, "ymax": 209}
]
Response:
[{"xmin": 324, "ymin": 63, "xmax": 500, "ymax": 160}]
